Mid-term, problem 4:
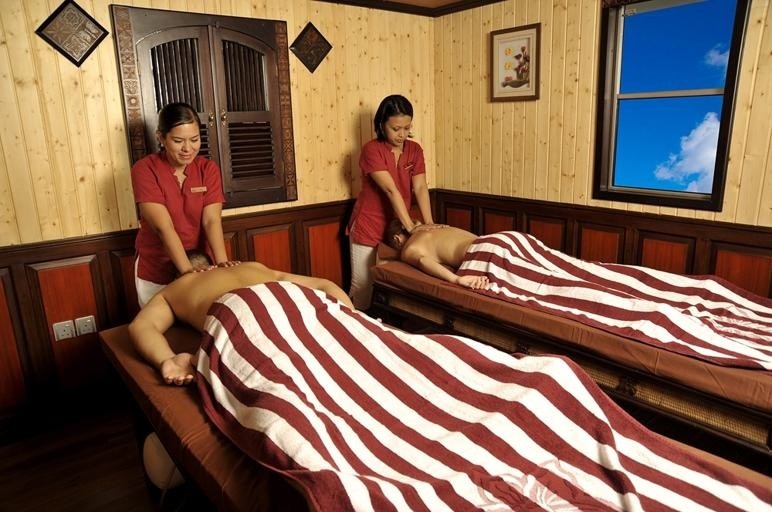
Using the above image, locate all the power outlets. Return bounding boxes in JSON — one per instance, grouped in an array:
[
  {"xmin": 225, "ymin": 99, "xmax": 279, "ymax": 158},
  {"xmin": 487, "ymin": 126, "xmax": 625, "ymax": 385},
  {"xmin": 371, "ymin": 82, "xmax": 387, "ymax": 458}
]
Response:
[{"xmin": 53, "ymin": 315, "xmax": 101, "ymax": 344}]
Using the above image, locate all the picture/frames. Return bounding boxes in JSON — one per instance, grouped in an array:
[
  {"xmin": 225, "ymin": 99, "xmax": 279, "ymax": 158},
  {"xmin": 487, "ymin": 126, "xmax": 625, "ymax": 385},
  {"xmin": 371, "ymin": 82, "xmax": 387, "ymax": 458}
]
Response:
[
  {"xmin": 289, "ymin": 20, "xmax": 333, "ymax": 75},
  {"xmin": 488, "ymin": 21, "xmax": 543, "ymax": 102},
  {"xmin": 34, "ymin": 0, "xmax": 109, "ymax": 68}
]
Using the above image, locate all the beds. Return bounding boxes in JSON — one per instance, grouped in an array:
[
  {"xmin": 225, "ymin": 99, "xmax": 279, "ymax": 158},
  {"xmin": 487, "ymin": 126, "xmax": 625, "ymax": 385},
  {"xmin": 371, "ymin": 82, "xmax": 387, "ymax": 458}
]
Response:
[
  {"xmin": 99, "ymin": 316, "xmax": 772, "ymax": 512},
  {"xmin": 370, "ymin": 262, "xmax": 772, "ymax": 452}
]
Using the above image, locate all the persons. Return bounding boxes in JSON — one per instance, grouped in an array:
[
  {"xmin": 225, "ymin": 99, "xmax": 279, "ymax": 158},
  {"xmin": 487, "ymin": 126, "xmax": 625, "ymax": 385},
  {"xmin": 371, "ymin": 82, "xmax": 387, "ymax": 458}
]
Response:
[
  {"xmin": 131, "ymin": 100, "xmax": 242, "ymax": 310},
  {"xmin": 384, "ymin": 218, "xmax": 490, "ymax": 290},
  {"xmin": 127, "ymin": 250, "xmax": 358, "ymax": 387},
  {"xmin": 344, "ymin": 94, "xmax": 451, "ymax": 313}
]
